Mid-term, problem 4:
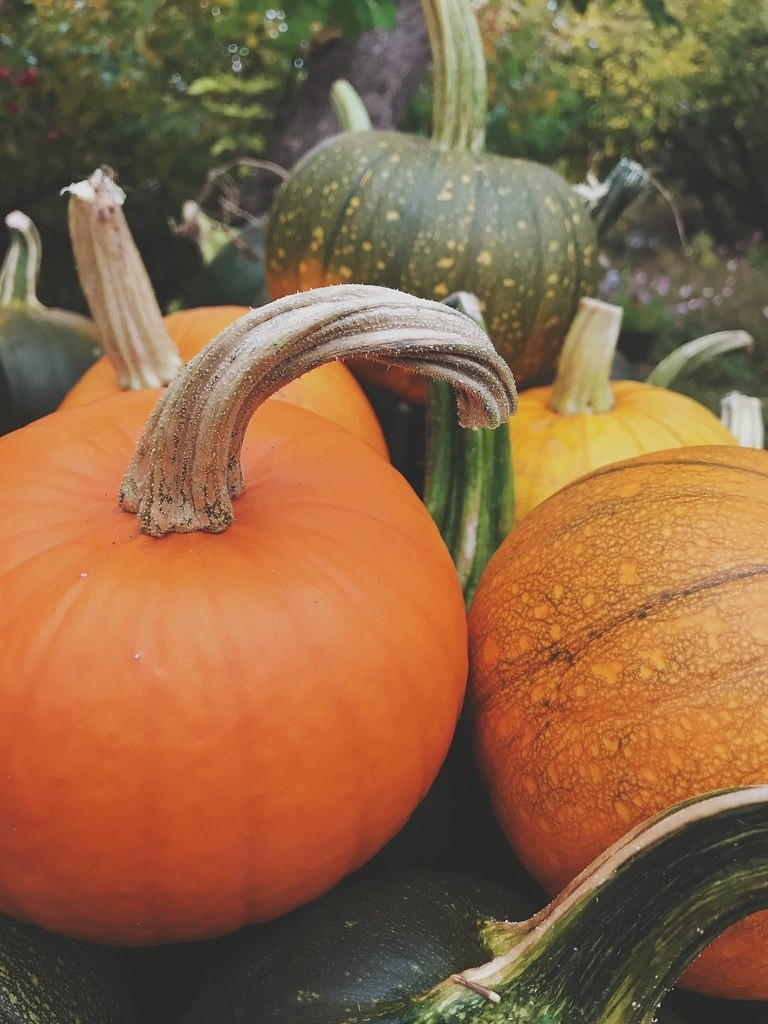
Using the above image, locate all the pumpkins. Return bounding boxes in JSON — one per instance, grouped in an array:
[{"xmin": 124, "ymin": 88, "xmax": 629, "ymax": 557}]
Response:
[{"xmin": 0, "ymin": 0, "xmax": 768, "ymax": 1003}]
